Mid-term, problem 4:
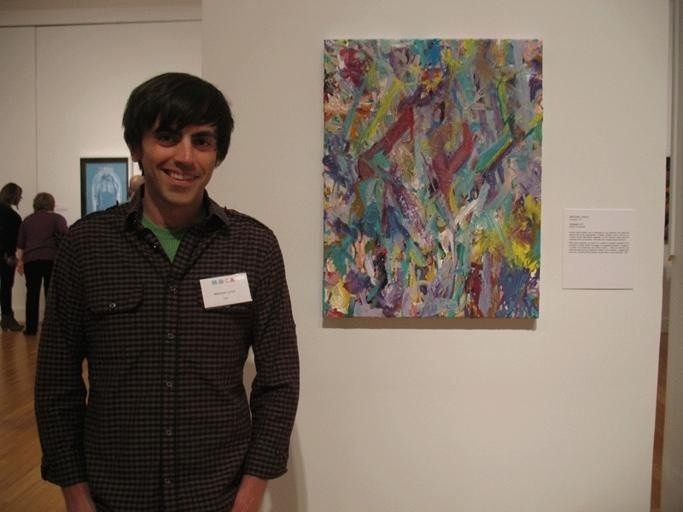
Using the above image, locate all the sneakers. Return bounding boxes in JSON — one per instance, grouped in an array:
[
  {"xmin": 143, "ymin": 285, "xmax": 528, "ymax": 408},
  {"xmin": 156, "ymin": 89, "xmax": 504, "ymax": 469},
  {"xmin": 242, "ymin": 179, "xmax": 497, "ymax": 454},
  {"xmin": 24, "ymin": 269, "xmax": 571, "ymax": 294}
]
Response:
[{"xmin": 24, "ymin": 330, "xmax": 37, "ymax": 336}]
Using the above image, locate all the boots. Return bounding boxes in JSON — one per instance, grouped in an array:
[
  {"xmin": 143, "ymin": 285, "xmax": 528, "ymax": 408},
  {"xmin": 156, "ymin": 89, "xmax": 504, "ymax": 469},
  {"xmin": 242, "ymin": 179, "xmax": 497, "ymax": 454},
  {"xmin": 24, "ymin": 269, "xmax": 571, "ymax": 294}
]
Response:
[{"xmin": 0, "ymin": 311, "xmax": 24, "ymax": 332}]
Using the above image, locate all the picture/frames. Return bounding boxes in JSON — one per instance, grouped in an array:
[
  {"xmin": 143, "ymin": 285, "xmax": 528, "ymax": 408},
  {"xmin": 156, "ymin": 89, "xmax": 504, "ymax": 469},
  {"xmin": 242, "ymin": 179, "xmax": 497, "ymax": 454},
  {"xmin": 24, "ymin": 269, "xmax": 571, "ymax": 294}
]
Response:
[{"xmin": 80, "ymin": 158, "xmax": 128, "ymax": 217}]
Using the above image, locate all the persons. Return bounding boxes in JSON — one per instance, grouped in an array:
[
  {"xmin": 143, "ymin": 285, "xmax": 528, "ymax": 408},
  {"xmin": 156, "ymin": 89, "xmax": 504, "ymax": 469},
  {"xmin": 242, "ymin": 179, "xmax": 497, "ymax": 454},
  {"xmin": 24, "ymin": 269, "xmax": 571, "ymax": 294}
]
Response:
[
  {"xmin": 0, "ymin": 182, "xmax": 25, "ymax": 332},
  {"xmin": 34, "ymin": 72, "xmax": 300, "ymax": 512},
  {"xmin": 16, "ymin": 192, "xmax": 68, "ymax": 336},
  {"xmin": 128, "ymin": 176, "xmax": 145, "ymax": 203}
]
